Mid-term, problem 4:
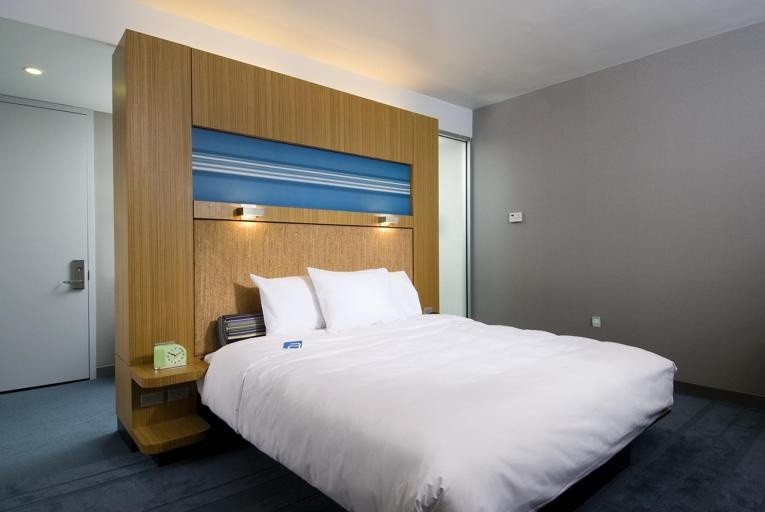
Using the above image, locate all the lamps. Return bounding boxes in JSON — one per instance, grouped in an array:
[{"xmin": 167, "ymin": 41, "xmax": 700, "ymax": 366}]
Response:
[
  {"xmin": 235, "ymin": 205, "xmax": 265, "ymax": 219},
  {"xmin": 378, "ymin": 215, "xmax": 400, "ymax": 225}
]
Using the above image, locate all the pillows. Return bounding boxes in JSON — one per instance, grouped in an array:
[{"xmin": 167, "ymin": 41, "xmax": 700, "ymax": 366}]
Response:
[
  {"xmin": 249, "ymin": 273, "xmax": 323, "ymax": 336},
  {"xmin": 387, "ymin": 270, "xmax": 422, "ymax": 318},
  {"xmin": 308, "ymin": 265, "xmax": 401, "ymax": 329}
]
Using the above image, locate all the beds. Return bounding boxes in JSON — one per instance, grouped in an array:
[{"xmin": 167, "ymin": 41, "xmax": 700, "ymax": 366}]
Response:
[{"xmin": 194, "ymin": 219, "xmax": 678, "ymax": 512}]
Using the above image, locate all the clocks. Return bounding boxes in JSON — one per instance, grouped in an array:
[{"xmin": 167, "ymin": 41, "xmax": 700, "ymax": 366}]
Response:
[{"xmin": 152, "ymin": 341, "xmax": 186, "ymax": 368}]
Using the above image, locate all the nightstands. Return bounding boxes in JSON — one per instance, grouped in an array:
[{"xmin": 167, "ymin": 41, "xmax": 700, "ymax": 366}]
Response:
[{"xmin": 113, "ymin": 357, "xmax": 210, "ymax": 455}]
